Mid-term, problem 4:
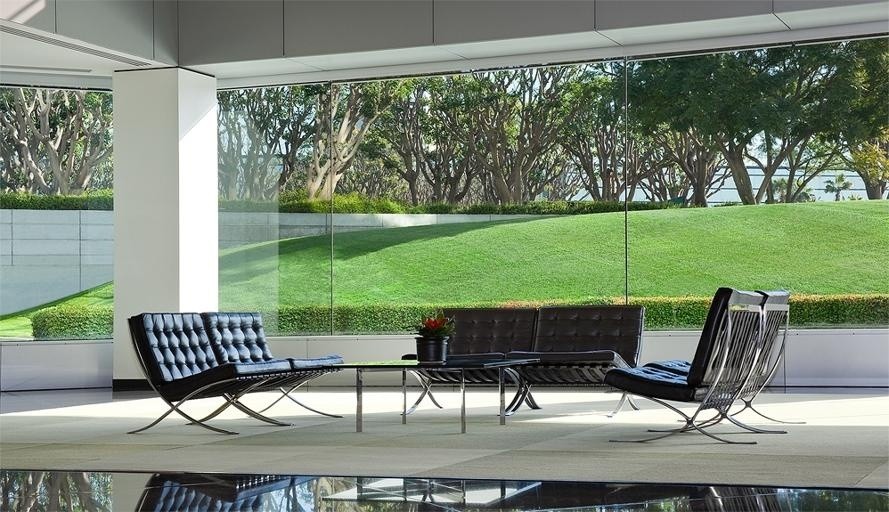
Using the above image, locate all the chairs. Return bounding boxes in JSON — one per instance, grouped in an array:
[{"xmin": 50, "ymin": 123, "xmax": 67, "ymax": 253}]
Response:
[
  {"xmin": 604, "ymin": 286, "xmax": 807, "ymax": 446},
  {"xmin": 401, "ymin": 304, "xmax": 646, "ymax": 418},
  {"xmin": 128, "ymin": 312, "xmax": 346, "ymax": 434},
  {"xmin": 134, "ymin": 475, "xmax": 792, "ymax": 512}
]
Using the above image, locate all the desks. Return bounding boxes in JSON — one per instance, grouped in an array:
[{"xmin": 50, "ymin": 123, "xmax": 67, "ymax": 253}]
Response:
[{"xmin": 331, "ymin": 358, "xmax": 541, "ymax": 434}]
